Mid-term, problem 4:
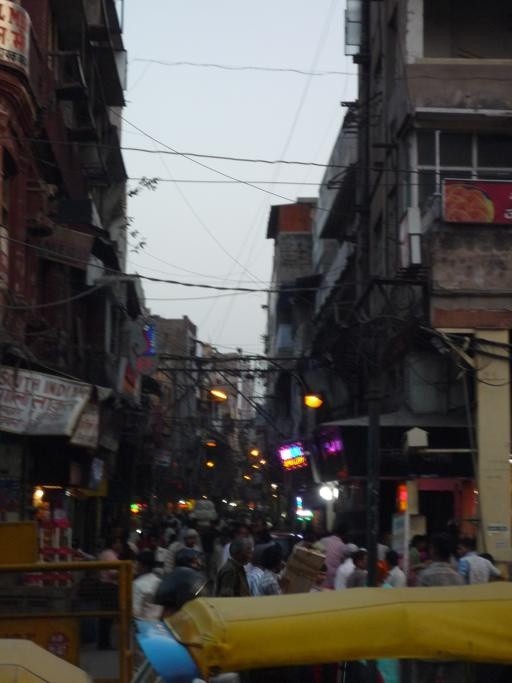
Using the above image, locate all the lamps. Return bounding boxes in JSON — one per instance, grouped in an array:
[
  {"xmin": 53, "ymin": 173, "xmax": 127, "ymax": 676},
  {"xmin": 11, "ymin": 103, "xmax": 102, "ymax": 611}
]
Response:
[
  {"xmin": 319, "ymin": 482, "xmax": 339, "ymax": 501},
  {"xmin": 202, "ymin": 438, "xmax": 267, "ymax": 482},
  {"xmin": 304, "ymin": 392, "xmax": 322, "ymax": 410}
]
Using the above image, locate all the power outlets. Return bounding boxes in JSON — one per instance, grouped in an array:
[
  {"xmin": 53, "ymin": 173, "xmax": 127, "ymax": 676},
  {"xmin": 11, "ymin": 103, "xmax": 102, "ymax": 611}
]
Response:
[{"xmin": 153, "ymin": 379, "xmax": 227, "ymax": 401}]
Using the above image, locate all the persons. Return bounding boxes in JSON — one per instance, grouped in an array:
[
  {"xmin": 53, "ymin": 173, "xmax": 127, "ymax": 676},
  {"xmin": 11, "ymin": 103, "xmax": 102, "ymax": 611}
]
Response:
[{"xmin": 94, "ymin": 501, "xmax": 512, "ymax": 681}]
